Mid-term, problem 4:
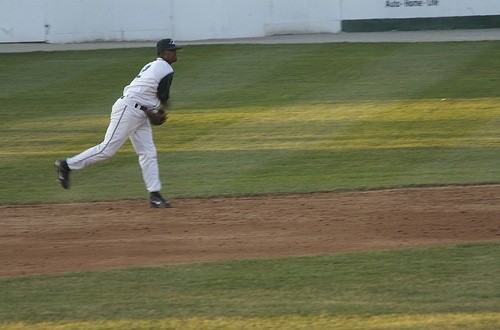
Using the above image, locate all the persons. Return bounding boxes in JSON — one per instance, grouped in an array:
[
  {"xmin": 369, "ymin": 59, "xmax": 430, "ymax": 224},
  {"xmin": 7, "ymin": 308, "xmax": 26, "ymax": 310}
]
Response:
[{"xmin": 53, "ymin": 36, "xmax": 185, "ymax": 211}]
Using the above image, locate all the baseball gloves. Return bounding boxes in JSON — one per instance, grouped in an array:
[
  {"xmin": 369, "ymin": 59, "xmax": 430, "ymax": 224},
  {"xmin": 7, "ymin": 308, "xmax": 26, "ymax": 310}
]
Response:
[{"xmin": 151, "ymin": 109, "xmax": 167, "ymax": 125}]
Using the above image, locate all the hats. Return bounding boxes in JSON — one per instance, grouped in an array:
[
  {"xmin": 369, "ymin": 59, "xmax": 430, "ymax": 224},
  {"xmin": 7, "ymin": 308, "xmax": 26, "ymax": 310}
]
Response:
[{"xmin": 157, "ymin": 38, "xmax": 182, "ymax": 51}]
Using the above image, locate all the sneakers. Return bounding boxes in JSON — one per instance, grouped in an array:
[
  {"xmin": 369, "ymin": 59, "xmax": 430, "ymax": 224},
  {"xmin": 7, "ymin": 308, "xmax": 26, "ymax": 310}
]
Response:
[
  {"xmin": 55, "ymin": 160, "xmax": 68, "ymax": 189},
  {"xmin": 149, "ymin": 196, "xmax": 171, "ymax": 208}
]
